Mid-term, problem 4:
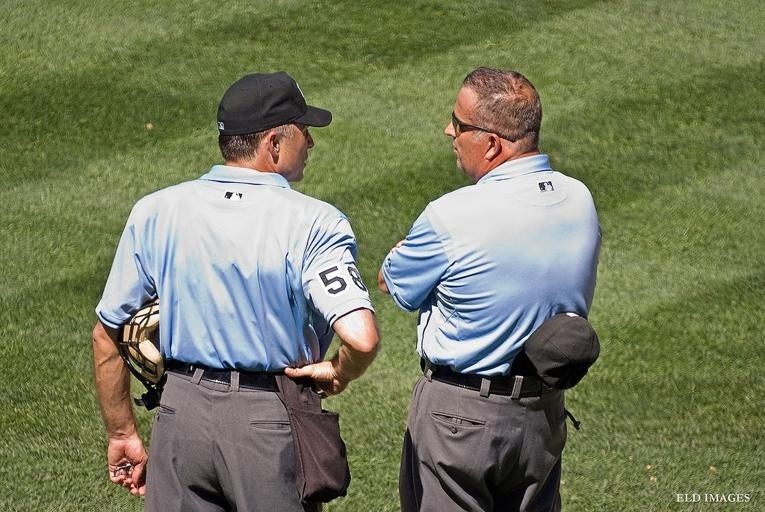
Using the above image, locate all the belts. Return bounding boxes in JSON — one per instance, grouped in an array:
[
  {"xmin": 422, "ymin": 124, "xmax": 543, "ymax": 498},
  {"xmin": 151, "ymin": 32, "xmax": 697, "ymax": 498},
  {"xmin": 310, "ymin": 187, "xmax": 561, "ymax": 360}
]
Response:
[
  {"xmin": 419, "ymin": 359, "xmax": 540, "ymax": 403},
  {"xmin": 160, "ymin": 359, "xmax": 279, "ymax": 394}
]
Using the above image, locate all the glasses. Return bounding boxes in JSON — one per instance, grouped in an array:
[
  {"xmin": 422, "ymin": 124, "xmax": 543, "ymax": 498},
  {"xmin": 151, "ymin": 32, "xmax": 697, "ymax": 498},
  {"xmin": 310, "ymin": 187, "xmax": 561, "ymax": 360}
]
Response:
[{"xmin": 450, "ymin": 109, "xmax": 518, "ymax": 145}]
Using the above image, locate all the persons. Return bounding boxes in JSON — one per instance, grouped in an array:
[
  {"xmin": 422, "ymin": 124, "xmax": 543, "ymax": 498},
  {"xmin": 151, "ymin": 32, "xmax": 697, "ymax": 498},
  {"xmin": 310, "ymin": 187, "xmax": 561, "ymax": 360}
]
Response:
[
  {"xmin": 376, "ymin": 66, "xmax": 604, "ymax": 512},
  {"xmin": 85, "ymin": 71, "xmax": 380, "ymax": 512}
]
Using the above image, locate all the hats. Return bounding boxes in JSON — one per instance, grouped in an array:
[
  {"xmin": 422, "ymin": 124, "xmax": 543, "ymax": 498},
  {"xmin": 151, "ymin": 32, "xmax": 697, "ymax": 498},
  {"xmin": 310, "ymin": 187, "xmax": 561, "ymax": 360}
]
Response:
[
  {"xmin": 506, "ymin": 309, "xmax": 601, "ymax": 401},
  {"xmin": 216, "ymin": 71, "xmax": 333, "ymax": 135}
]
